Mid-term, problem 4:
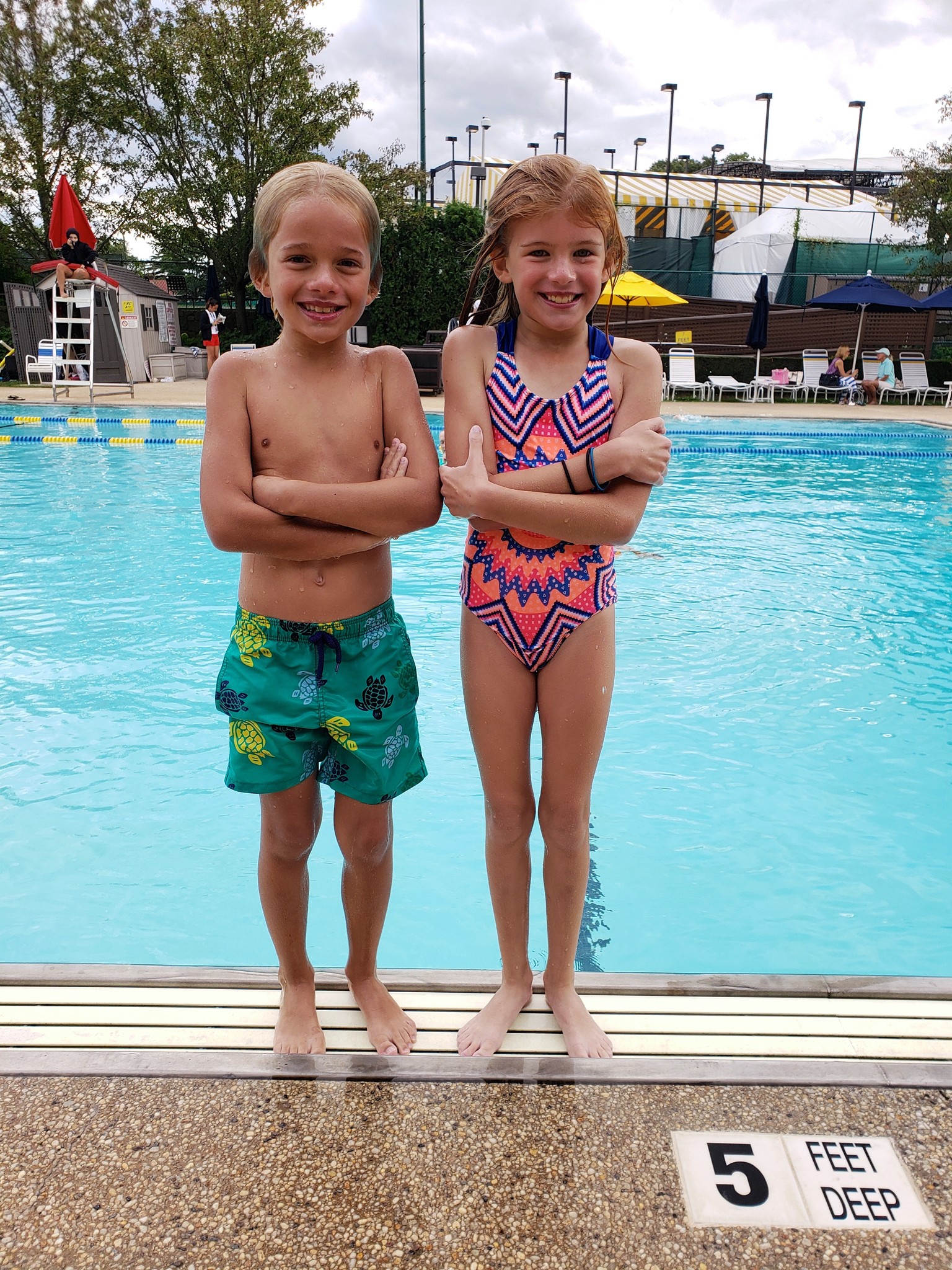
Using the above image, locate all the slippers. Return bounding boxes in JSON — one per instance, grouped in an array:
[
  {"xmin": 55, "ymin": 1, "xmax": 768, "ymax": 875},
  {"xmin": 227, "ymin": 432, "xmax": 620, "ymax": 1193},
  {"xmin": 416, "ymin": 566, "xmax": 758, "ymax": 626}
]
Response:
[{"xmin": 8, "ymin": 395, "xmax": 25, "ymax": 400}]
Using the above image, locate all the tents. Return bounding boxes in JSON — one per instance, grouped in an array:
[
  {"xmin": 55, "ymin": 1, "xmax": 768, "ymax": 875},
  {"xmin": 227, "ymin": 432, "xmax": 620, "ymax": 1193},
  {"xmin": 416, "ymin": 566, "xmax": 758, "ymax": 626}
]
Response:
[{"xmin": 711, "ymin": 195, "xmax": 952, "ymax": 305}]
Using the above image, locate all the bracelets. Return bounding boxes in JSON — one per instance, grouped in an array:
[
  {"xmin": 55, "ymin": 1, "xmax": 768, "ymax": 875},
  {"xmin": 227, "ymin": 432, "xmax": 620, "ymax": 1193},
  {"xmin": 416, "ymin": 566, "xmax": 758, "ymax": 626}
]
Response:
[
  {"xmin": 561, "ymin": 459, "xmax": 578, "ymax": 495},
  {"xmin": 586, "ymin": 447, "xmax": 610, "ymax": 493}
]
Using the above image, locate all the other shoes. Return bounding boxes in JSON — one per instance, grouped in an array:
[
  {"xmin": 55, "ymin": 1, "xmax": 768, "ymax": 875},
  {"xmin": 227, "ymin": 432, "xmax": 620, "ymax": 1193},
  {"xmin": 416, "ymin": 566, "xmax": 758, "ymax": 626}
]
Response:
[
  {"xmin": 839, "ymin": 398, "xmax": 848, "ymax": 405},
  {"xmin": 857, "ymin": 400, "xmax": 866, "ymax": 406}
]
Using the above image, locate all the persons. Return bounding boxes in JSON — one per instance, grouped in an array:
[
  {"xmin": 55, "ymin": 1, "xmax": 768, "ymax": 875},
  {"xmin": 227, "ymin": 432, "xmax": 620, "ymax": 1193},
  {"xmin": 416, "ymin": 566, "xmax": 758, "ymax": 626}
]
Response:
[
  {"xmin": 56, "ymin": 227, "xmax": 99, "ymax": 298},
  {"xmin": 199, "ymin": 297, "xmax": 220, "ymax": 372},
  {"xmin": 439, "ymin": 155, "xmax": 672, "ymax": 1056},
  {"xmin": 439, "ymin": 430, "xmax": 447, "ymax": 463},
  {"xmin": 825, "ymin": 345, "xmax": 858, "ymax": 404},
  {"xmin": 202, "ymin": 162, "xmax": 445, "ymax": 1056},
  {"xmin": 861, "ymin": 348, "xmax": 895, "ymax": 404}
]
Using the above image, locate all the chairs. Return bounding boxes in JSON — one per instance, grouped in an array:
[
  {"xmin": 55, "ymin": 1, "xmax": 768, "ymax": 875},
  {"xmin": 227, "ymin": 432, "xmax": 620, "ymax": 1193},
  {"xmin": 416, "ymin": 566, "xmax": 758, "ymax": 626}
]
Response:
[
  {"xmin": 231, "ymin": 344, "xmax": 256, "ymax": 351},
  {"xmin": 26, "ymin": 339, "xmax": 63, "ymax": 385},
  {"xmin": 662, "ymin": 348, "xmax": 952, "ymax": 408}
]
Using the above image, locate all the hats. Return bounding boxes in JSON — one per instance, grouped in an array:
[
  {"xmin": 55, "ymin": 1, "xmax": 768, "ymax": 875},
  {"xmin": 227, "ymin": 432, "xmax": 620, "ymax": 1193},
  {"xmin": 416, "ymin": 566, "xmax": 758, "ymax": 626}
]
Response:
[{"xmin": 875, "ymin": 348, "xmax": 890, "ymax": 357}]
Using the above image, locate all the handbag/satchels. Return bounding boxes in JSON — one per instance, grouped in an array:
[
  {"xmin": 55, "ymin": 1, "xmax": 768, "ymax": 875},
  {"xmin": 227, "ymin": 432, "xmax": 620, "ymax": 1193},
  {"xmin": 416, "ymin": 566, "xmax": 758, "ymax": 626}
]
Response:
[
  {"xmin": 788, "ymin": 371, "xmax": 803, "ymax": 385},
  {"xmin": 771, "ymin": 368, "xmax": 789, "ymax": 384},
  {"xmin": 819, "ymin": 373, "xmax": 840, "ymax": 386}
]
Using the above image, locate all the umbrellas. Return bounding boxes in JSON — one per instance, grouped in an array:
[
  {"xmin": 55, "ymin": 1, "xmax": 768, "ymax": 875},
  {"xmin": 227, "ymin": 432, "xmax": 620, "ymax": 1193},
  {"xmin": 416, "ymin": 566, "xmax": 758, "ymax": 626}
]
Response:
[
  {"xmin": 919, "ymin": 285, "xmax": 952, "ymax": 310},
  {"xmin": 745, "ymin": 269, "xmax": 770, "ymax": 402},
  {"xmin": 597, "ymin": 271, "xmax": 689, "ymax": 338},
  {"xmin": 801, "ymin": 270, "xmax": 930, "ymax": 403},
  {"xmin": 49, "ymin": 174, "xmax": 97, "ymax": 251}
]
